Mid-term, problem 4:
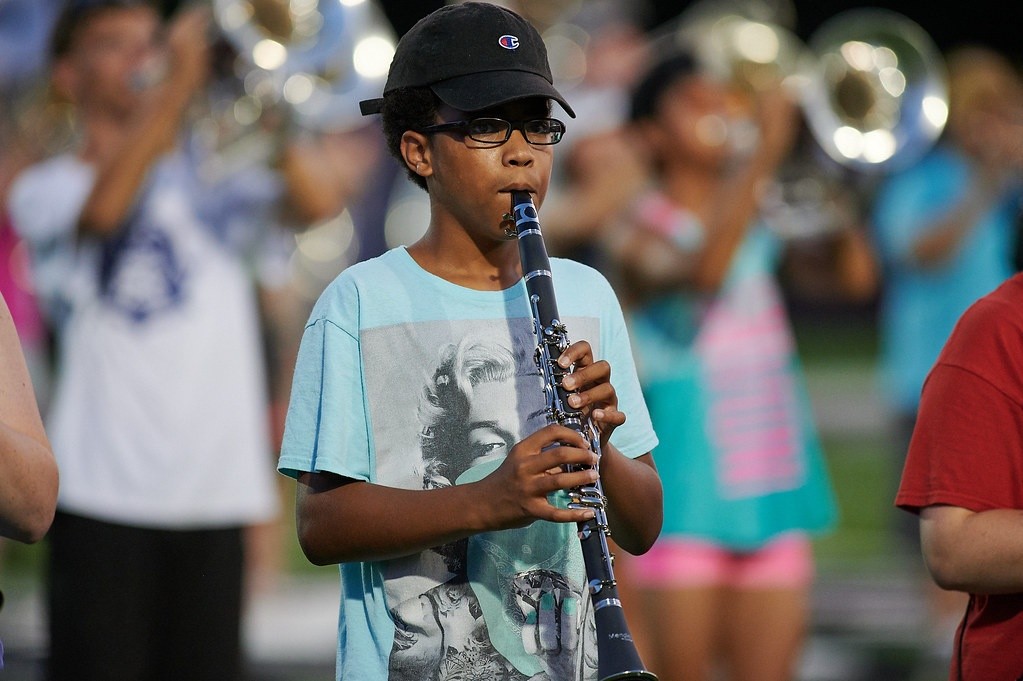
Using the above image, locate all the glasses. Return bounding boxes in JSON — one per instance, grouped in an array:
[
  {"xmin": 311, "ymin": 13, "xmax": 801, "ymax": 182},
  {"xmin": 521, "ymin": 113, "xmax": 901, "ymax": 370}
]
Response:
[{"xmin": 415, "ymin": 117, "xmax": 566, "ymax": 145}]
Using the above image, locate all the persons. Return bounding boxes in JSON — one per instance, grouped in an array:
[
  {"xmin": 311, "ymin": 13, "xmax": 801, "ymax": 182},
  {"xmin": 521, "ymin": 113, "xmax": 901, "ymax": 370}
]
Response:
[
  {"xmin": 3, "ymin": 1, "xmax": 274, "ymax": 681},
  {"xmin": 387, "ymin": 329, "xmax": 599, "ymax": 681},
  {"xmin": 874, "ymin": 46, "xmax": 1021, "ymax": 408},
  {"xmin": 599, "ymin": 50, "xmax": 870, "ymax": 681},
  {"xmin": 1, "ymin": 295, "xmax": 59, "ymax": 546},
  {"xmin": 275, "ymin": 4, "xmax": 663, "ymax": 680},
  {"xmin": 896, "ymin": 268, "xmax": 1023, "ymax": 681}
]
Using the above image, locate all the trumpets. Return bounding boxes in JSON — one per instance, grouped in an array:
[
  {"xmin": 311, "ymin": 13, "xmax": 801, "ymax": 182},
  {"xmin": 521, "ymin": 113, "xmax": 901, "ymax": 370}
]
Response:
[
  {"xmin": 728, "ymin": 9, "xmax": 959, "ymax": 179},
  {"xmin": 135, "ymin": 1, "xmax": 402, "ymax": 173}
]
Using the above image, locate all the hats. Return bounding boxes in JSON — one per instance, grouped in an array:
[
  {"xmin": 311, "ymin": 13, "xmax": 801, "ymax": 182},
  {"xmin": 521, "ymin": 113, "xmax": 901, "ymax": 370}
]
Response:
[{"xmin": 358, "ymin": 2, "xmax": 577, "ymax": 120}]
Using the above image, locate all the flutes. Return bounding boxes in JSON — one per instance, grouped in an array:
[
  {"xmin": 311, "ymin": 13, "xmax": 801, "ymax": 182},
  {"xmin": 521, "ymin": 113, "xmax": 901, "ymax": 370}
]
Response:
[{"xmin": 494, "ymin": 188, "xmax": 667, "ymax": 681}]
